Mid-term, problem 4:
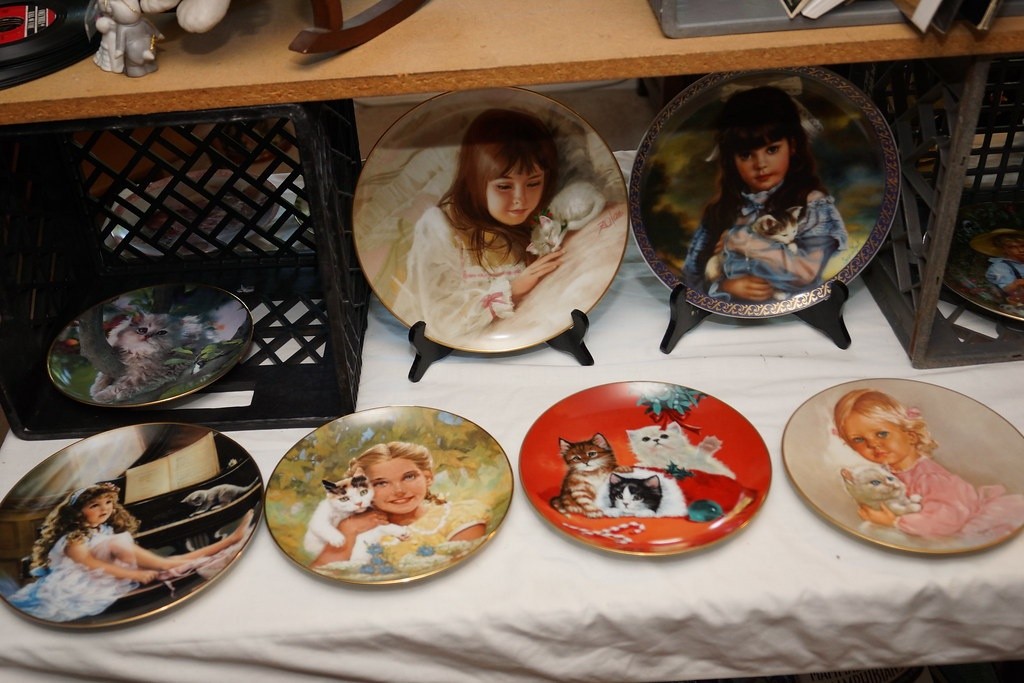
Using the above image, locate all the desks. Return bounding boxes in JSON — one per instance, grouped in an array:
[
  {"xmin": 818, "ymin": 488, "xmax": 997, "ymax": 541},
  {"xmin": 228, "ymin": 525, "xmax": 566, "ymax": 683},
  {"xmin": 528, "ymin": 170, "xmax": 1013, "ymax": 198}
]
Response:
[{"xmin": 0, "ymin": 140, "xmax": 1024, "ymax": 681}]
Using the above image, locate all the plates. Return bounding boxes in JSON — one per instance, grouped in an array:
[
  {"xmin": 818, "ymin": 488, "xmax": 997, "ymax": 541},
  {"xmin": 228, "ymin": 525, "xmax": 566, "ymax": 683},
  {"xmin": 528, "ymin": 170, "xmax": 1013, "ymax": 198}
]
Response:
[
  {"xmin": 350, "ymin": 87, "xmax": 628, "ymax": 354},
  {"xmin": 629, "ymin": 67, "xmax": 900, "ymax": 320},
  {"xmin": 1, "ymin": 422, "xmax": 264, "ymax": 628},
  {"xmin": 110, "ymin": 169, "xmax": 282, "ymax": 256},
  {"xmin": 517, "ymin": 382, "xmax": 773, "ymax": 556},
  {"xmin": 266, "ymin": 405, "xmax": 514, "ymax": 584},
  {"xmin": 922, "ymin": 201, "xmax": 1024, "ymax": 322},
  {"xmin": 781, "ymin": 378, "xmax": 1024, "ymax": 553},
  {"xmin": 47, "ymin": 285, "xmax": 254, "ymax": 407},
  {"xmin": 295, "ymin": 159, "xmax": 364, "ymax": 235}
]
